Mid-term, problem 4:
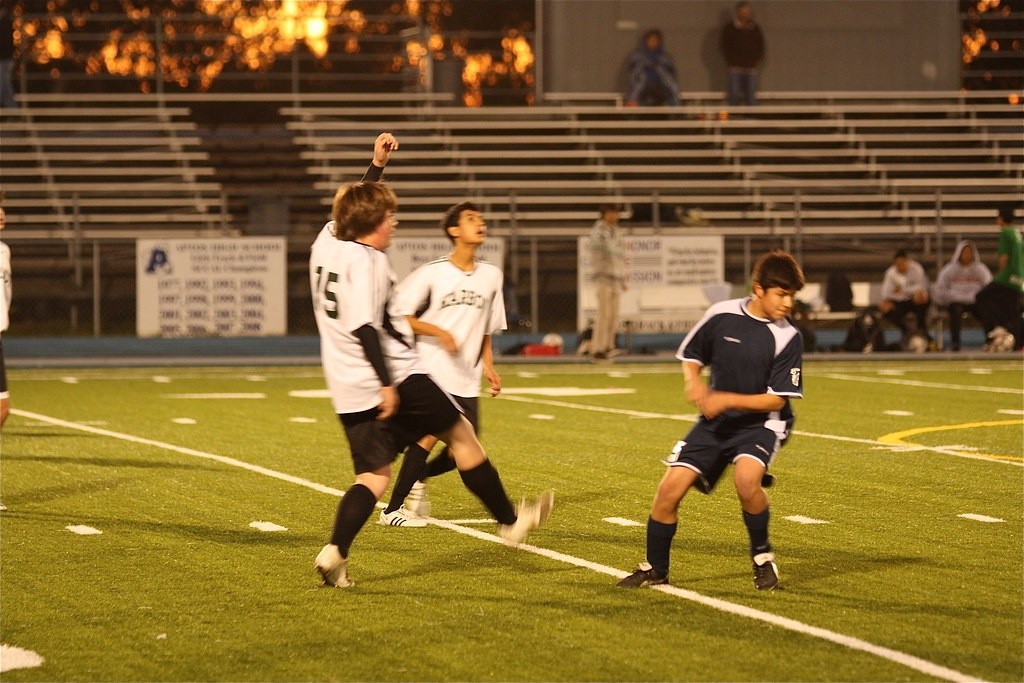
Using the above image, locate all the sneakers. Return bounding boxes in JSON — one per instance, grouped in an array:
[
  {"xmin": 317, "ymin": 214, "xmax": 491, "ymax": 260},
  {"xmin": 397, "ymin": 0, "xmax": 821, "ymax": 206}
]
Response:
[
  {"xmin": 616, "ymin": 562, "xmax": 669, "ymax": 589},
  {"xmin": 499, "ymin": 491, "xmax": 555, "ymax": 543},
  {"xmin": 380, "ymin": 504, "xmax": 427, "ymax": 528},
  {"xmin": 314, "ymin": 544, "xmax": 355, "ymax": 588},
  {"xmin": 404, "ymin": 480, "xmax": 426, "ymax": 510},
  {"xmin": 752, "ymin": 550, "xmax": 779, "ymax": 591}
]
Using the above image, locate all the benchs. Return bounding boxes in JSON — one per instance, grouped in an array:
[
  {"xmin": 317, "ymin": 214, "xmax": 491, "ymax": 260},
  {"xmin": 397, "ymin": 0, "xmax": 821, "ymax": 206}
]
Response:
[{"xmin": 0, "ymin": 87, "xmax": 1020, "ymax": 350}]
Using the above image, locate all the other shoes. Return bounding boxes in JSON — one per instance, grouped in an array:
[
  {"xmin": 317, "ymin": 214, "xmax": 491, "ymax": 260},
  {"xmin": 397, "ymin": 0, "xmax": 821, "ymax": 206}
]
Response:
[{"xmin": 591, "ymin": 353, "xmax": 610, "ymax": 362}]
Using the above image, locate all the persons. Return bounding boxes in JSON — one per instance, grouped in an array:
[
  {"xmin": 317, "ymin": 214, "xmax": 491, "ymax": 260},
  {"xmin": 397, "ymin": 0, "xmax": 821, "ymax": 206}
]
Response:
[
  {"xmin": 974, "ymin": 200, "xmax": 1024, "ymax": 353},
  {"xmin": 375, "ymin": 201, "xmax": 509, "ymax": 526},
  {"xmin": 720, "ymin": 0, "xmax": 766, "ymax": 105},
  {"xmin": 618, "ymin": 252, "xmax": 803, "ymax": 588},
  {"xmin": 310, "ymin": 132, "xmax": 553, "ymax": 587},
  {"xmin": 0, "ymin": 208, "xmax": 13, "ymax": 427},
  {"xmin": 936, "ymin": 239, "xmax": 995, "ymax": 353},
  {"xmin": 586, "ymin": 202, "xmax": 629, "ymax": 358},
  {"xmin": 878, "ymin": 248, "xmax": 934, "ymax": 352},
  {"xmin": 625, "ymin": 29, "xmax": 680, "ymax": 106}
]
